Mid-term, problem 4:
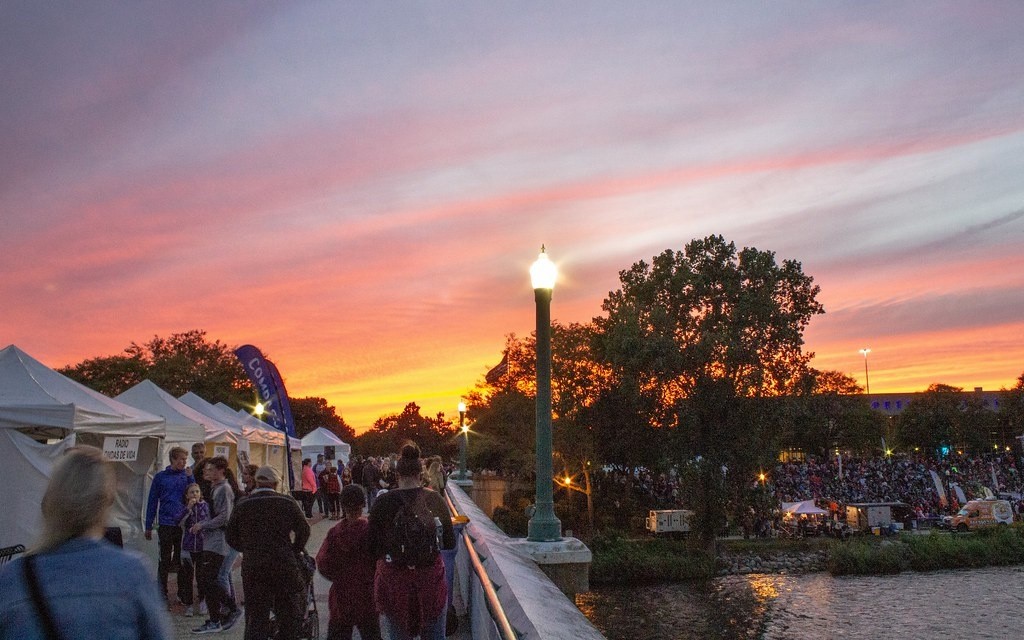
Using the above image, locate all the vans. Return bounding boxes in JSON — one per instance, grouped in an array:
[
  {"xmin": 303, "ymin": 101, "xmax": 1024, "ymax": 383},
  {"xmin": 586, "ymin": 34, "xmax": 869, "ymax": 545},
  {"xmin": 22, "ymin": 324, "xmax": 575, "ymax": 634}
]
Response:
[{"xmin": 949, "ymin": 498, "xmax": 1013, "ymax": 532}]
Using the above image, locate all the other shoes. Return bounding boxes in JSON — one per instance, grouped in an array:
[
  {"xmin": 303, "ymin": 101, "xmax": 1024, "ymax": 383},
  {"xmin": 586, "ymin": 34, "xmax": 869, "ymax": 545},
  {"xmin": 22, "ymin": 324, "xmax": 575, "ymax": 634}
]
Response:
[
  {"xmin": 185, "ymin": 606, "xmax": 195, "ymax": 617},
  {"xmin": 306, "ymin": 513, "xmax": 313, "ymax": 518},
  {"xmin": 319, "ymin": 509, "xmax": 346, "ymax": 520},
  {"xmin": 199, "ymin": 602, "xmax": 208, "ymax": 615}
]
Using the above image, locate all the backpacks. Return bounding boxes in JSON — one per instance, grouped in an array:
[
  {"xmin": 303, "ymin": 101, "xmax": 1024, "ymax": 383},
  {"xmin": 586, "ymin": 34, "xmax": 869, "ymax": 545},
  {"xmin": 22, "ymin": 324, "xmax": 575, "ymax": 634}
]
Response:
[
  {"xmin": 327, "ymin": 474, "xmax": 340, "ymax": 494},
  {"xmin": 383, "ymin": 487, "xmax": 437, "ymax": 566}
]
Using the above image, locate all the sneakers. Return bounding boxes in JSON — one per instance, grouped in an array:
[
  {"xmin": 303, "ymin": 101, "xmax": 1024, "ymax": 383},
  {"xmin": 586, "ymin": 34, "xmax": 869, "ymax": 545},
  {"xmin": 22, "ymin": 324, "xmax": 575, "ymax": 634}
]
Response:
[
  {"xmin": 223, "ymin": 609, "xmax": 244, "ymax": 630},
  {"xmin": 192, "ymin": 620, "xmax": 221, "ymax": 633}
]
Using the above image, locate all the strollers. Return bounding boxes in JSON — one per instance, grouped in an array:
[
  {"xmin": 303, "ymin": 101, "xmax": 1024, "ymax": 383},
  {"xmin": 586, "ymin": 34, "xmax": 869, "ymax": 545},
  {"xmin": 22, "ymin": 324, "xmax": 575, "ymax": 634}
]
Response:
[{"xmin": 290, "ymin": 544, "xmax": 321, "ymax": 640}]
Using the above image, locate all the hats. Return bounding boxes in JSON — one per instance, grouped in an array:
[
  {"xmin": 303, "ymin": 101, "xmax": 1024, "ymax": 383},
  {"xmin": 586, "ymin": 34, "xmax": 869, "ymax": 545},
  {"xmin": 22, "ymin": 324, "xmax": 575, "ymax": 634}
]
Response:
[
  {"xmin": 367, "ymin": 457, "xmax": 375, "ymax": 462},
  {"xmin": 255, "ymin": 464, "xmax": 283, "ymax": 482}
]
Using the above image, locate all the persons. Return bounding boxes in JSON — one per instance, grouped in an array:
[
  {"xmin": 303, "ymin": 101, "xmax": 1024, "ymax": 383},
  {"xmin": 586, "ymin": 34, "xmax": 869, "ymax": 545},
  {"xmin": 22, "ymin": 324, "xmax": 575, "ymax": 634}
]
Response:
[
  {"xmin": 313, "ymin": 483, "xmax": 382, "ymax": 640},
  {"xmin": 303, "ymin": 433, "xmax": 1024, "ymax": 544},
  {"xmin": 226, "ymin": 463, "xmax": 314, "ymax": 640},
  {"xmin": 370, "ymin": 440, "xmax": 457, "ymax": 640},
  {"xmin": 143, "ymin": 445, "xmax": 260, "ymax": 631},
  {"xmin": 0, "ymin": 441, "xmax": 185, "ymax": 639}
]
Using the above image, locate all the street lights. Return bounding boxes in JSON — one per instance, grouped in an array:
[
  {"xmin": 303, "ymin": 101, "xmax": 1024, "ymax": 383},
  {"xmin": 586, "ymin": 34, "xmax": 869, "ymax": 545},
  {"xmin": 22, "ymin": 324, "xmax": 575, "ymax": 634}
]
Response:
[
  {"xmin": 946, "ymin": 469, "xmax": 951, "ymax": 499},
  {"xmin": 564, "ymin": 478, "xmax": 574, "ymax": 524},
  {"xmin": 859, "ymin": 348, "xmax": 871, "ymax": 397},
  {"xmin": 455, "ymin": 400, "xmax": 469, "ymax": 483},
  {"xmin": 759, "ymin": 473, "xmax": 774, "ymax": 530},
  {"xmin": 526, "ymin": 244, "xmax": 565, "ymax": 542}
]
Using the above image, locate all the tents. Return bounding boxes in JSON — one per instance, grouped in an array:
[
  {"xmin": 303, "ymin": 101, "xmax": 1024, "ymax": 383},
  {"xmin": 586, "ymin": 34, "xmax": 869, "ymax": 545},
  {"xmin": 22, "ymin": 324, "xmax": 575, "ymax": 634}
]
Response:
[{"xmin": 1, "ymin": 342, "xmax": 356, "ymax": 547}]
